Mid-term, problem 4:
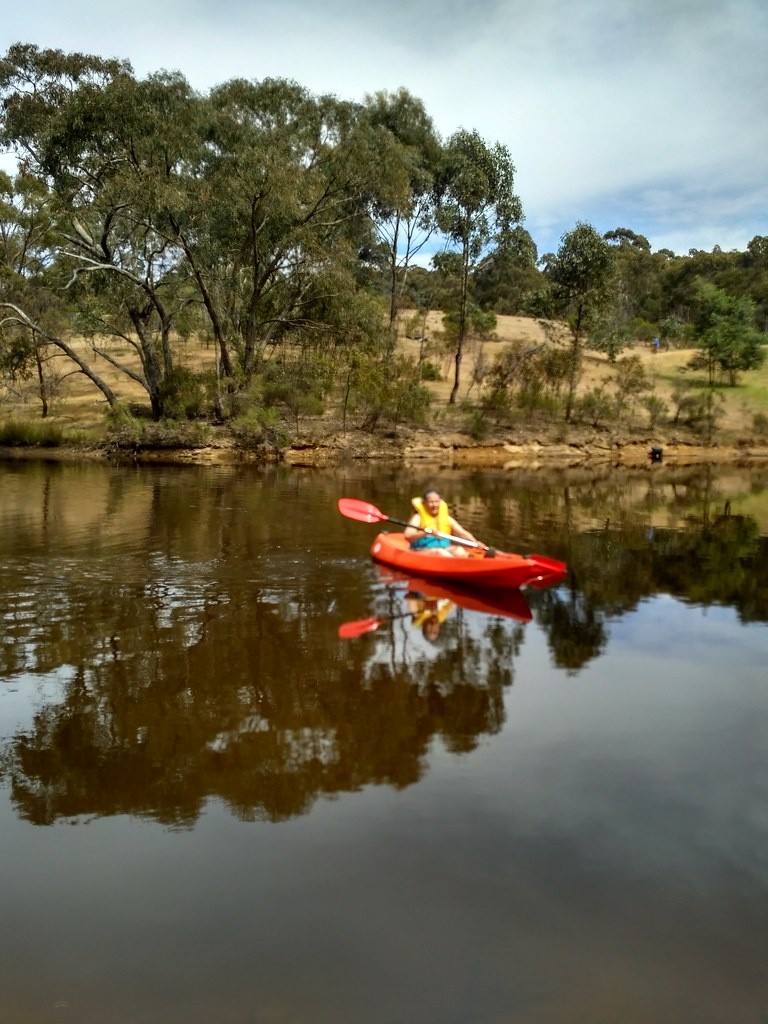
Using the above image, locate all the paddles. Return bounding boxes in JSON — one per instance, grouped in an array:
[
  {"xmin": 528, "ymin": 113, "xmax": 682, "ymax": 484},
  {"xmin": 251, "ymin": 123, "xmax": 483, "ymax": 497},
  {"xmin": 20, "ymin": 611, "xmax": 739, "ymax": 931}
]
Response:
[
  {"xmin": 338, "ymin": 610, "xmax": 423, "ymax": 640},
  {"xmin": 337, "ymin": 497, "xmax": 506, "ymax": 555}
]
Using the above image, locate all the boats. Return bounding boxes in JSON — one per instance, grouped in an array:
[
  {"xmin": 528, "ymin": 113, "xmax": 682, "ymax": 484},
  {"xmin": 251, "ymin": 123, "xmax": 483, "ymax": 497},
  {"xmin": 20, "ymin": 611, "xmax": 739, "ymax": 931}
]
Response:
[
  {"xmin": 375, "ymin": 564, "xmax": 533, "ymax": 624},
  {"xmin": 369, "ymin": 531, "xmax": 568, "ymax": 593}
]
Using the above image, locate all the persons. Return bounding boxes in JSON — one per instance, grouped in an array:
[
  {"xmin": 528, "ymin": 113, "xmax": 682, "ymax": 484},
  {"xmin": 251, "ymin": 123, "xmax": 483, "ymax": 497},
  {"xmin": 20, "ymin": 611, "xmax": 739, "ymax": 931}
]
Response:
[
  {"xmin": 652, "ymin": 336, "xmax": 659, "ymax": 354},
  {"xmin": 405, "ymin": 591, "xmax": 455, "ymax": 643},
  {"xmin": 404, "ymin": 489, "xmax": 486, "ymax": 558}
]
